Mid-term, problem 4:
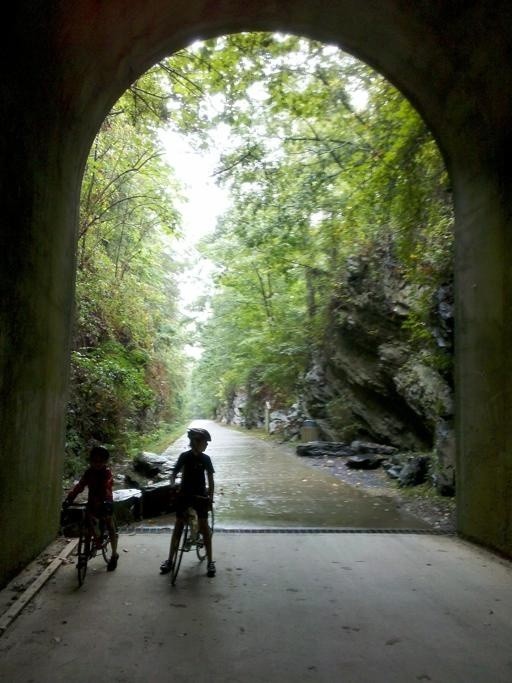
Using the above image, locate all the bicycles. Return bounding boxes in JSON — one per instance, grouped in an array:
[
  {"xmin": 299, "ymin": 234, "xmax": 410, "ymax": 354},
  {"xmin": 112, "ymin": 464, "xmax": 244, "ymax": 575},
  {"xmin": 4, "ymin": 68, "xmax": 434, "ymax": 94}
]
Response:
[
  {"xmin": 171, "ymin": 494, "xmax": 215, "ymax": 585},
  {"xmin": 70, "ymin": 501, "xmax": 112, "ymax": 585}
]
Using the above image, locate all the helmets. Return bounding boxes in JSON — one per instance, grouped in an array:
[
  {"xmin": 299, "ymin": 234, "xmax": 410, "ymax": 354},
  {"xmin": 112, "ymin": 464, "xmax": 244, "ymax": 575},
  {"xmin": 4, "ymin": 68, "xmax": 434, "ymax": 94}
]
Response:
[{"xmin": 187, "ymin": 428, "xmax": 212, "ymax": 442}]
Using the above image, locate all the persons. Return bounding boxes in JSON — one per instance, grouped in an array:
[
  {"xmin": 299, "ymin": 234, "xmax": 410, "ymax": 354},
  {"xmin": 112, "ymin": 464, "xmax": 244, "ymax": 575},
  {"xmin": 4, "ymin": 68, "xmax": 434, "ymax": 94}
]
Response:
[
  {"xmin": 158, "ymin": 427, "xmax": 219, "ymax": 574},
  {"xmin": 61, "ymin": 441, "xmax": 121, "ymax": 571}
]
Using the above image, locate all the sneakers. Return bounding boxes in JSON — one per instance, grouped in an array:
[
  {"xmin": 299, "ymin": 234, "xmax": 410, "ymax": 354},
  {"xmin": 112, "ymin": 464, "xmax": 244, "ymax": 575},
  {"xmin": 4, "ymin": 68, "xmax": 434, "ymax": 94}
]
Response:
[
  {"xmin": 207, "ymin": 562, "xmax": 216, "ymax": 572},
  {"xmin": 108, "ymin": 553, "xmax": 119, "ymax": 572},
  {"xmin": 160, "ymin": 560, "xmax": 174, "ymax": 570}
]
